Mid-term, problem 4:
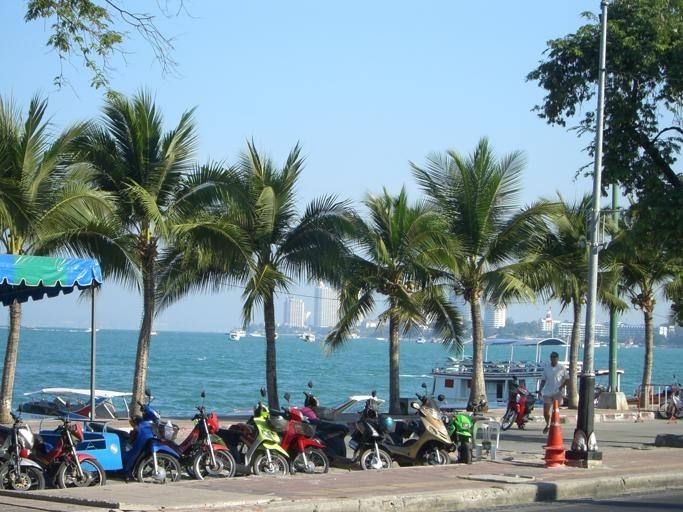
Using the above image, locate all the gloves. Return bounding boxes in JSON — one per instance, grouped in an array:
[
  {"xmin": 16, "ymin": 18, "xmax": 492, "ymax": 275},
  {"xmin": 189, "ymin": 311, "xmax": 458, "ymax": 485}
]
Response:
[
  {"xmin": 348, "ymin": 332, "xmax": 359, "ymax": 340},
  {"xmin": 299, "ymin": 332, "xmax": 316, "ymax": 342},
  {"xmin": 227, "ymin": 328, "xmax": 265, "ymax": 342},
  {"xmin": 427, "ymin": 338, "xmax": 626, "ymax": 407},
  {"xmin": 415, "ymin": 335, "xmax": 445, "ymax": 344}
]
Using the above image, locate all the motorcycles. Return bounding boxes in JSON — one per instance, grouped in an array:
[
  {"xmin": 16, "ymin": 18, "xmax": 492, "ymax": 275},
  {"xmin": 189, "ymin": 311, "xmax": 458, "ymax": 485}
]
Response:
[
  {"xmin": 264, "ymin": 392, "xmax": 330, "ymax": 474},
  {"xmin": 2, "ymin": 403, "xmax": 46, "ymax": 491},
  {"xmin": 501, "ymin": 375, "xmax": 539, "ymax": 430},
  {"xmin": 88, "ymin": 387, "xmax": 182, "ymax": 485},
  {"xmin": 302, "ymin": 382, "xmax": 320, "ymax": 407},
  {"xmin": 212, "ymin": 386, "xmax": 291, "ymax": 477},
  {"xmin": 307, "ymin": 390, "xmax": 393, "ymax": 470},
  {"xmin": 159, "ymin": 390, "xmax": 237, "ymax": 481},
  {"xmin": 26, "ymin": 399, "xmax": 107, "ymax": 490},
  {"xmin": 658, "ymin": 375, "xmax": 683, "ymax": 418},
  {"xmin": 377, "ymin": 383, "xmax": 487, "ymax": 467}
]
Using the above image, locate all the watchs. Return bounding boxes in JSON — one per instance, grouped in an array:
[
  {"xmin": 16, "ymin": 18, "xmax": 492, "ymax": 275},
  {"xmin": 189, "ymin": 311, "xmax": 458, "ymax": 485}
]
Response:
[
  {"xmin": 658, "ymin": 375, "xmax": 683, "ymax": 418},
  {"xmin": 501, "ymin": 375, "xmax": 539, "ymax": 430}
]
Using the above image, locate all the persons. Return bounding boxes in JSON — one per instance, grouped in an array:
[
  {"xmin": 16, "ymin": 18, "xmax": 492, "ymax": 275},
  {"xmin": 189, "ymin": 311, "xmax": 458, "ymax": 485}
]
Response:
[{"xmin": 538, "ymin": 351, "xmax": 570, "ymax": 434}]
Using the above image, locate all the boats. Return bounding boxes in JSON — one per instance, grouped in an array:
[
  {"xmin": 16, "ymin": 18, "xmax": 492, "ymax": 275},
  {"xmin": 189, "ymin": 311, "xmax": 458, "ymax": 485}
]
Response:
[
  {"xmin": 227, "ymin": 328, "xmax": 265, "ymax": 342},
  {"xmin": 299, "ymin": 332, "xmax": 316, "ymax": 342},
  {"xmin": 348, "ymin": 332, "xmax": 359, "ymax": 340},
  {"xmin": 415, "ymin": 335, "xmax": 445, "ymax": 344},
  {"xmin": 427, "ymin": 338, "xmax": 626, "ymax": 407}
]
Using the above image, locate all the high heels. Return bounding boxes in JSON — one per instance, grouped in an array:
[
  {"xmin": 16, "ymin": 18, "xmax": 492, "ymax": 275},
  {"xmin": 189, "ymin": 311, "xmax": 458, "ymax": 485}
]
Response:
[{"xmin": 543, "ymin": 400, "xmax": 568, "ymax": 466}]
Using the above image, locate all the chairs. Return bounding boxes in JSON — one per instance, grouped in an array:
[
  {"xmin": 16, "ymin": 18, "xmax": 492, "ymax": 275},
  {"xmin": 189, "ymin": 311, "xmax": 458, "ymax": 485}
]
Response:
[{"xmin": 543, "ymin": 424, "xmax": 550, "ymax": 434}]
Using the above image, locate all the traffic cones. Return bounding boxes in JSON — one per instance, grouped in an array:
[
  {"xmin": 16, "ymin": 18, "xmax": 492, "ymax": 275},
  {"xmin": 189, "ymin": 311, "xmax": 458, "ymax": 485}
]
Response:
[{"xmin": 543, "ymin": 400, "xmax": 568, "ymax": 466}]
